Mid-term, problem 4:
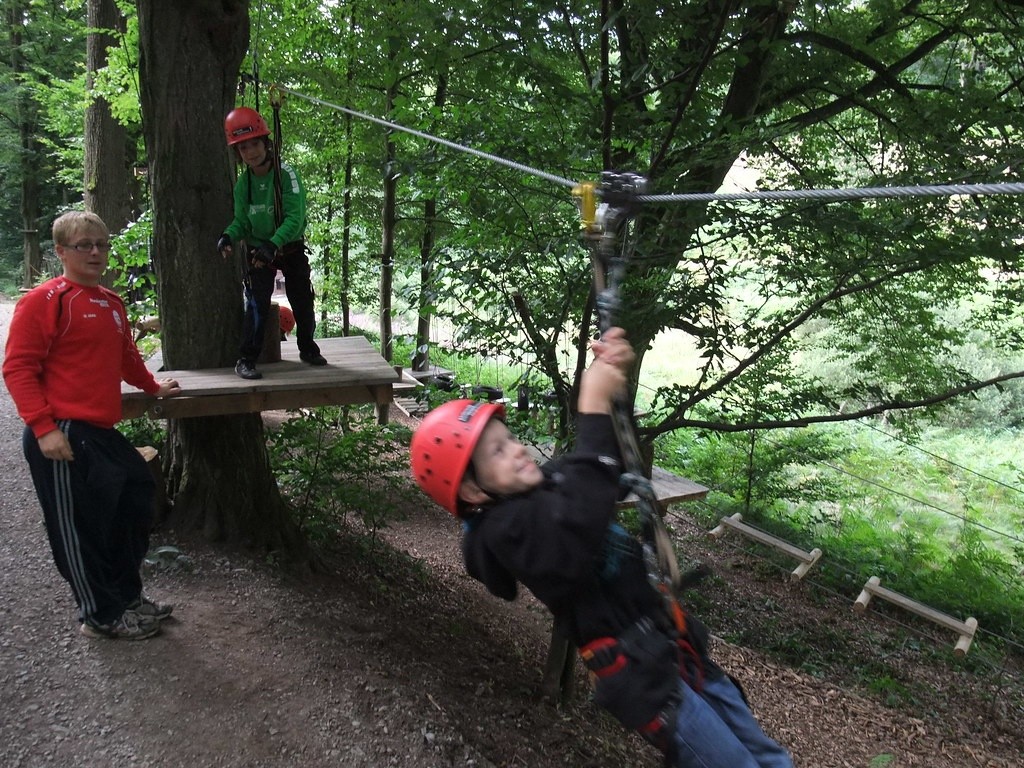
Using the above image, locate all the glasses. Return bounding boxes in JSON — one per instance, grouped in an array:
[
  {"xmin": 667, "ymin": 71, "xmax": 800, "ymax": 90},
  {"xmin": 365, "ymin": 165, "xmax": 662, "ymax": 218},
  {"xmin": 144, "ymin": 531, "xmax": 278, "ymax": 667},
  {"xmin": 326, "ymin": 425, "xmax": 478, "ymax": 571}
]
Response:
[{"xmin": 61, "ymin": 243, "xmax": 113, "ymax": 252}]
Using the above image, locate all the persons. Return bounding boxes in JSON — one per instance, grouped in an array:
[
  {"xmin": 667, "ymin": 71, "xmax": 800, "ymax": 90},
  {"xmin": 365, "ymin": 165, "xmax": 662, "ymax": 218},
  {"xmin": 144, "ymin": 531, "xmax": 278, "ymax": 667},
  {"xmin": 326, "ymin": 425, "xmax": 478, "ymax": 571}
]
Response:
[
  {"xmin": 2, "ymin": 210, "xmax": 182, "ymax": 640},
  {"xmin": 216, "ymin": 107, "xmax": 328, "ymax": 379},
  {"xmin": 410, "ymin": 328, "xmax": 796, "ymax": 768}
]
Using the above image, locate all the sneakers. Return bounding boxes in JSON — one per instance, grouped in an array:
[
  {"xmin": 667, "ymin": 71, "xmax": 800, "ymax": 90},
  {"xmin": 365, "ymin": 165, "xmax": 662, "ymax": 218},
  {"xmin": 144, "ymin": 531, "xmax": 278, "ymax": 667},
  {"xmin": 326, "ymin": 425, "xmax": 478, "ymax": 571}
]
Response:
[
  {"xmin": 80, "ymin": 609, "xmax": 161, "ymax": 640},
  {"xmin": 234, "ymin": 358, "xmax": 262, "ymax": 379},
  {"xmin": 299, "ymin": 352, "xmax": 327, "ymax": 365},
  {"xmin": 127, "ymin": 590, "xmax": 173, "ymax": 620}
]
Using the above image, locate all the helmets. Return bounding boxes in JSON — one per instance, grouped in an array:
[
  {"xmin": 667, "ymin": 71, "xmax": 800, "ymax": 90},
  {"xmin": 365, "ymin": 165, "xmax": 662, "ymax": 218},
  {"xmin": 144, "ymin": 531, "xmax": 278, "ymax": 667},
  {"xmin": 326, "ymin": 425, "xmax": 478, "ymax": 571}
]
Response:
[
  {"xmin": 410, "ymin": 399, "xmax": 506, "ymax": 516},
  {"xmin": 279, "ymin": 306, "xmax": 295, "ymax": 333},
  {"xmin": 225, "ymin": 107, "xmax": 271, "ymax": 153}
]
